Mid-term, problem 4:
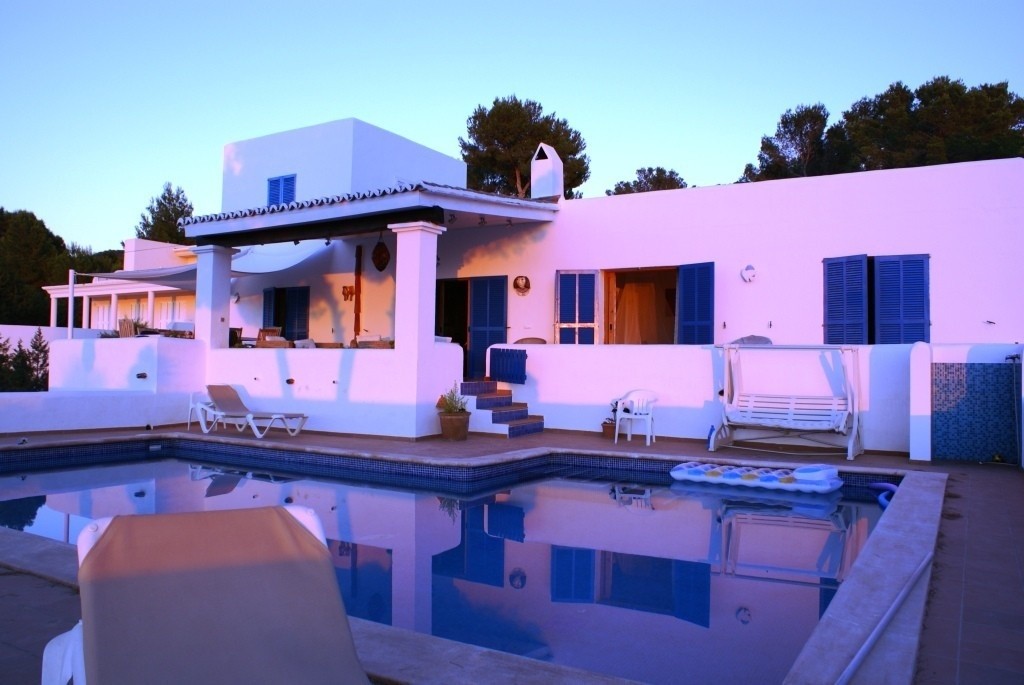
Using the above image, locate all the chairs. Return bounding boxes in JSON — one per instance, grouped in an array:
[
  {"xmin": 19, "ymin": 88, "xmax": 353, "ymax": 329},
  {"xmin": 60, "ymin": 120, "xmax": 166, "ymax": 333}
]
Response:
[
  {"xmin": 256, "ymin": 328, "xmax": 279, "ymax": 347},
  {"xmin": 613, "ymin": 388, "xmax": 660, "ymax": 446},
  {"xmin": 192, "ymin": 382, "xmax": 309, "ymax": 438},
  {"xmin": 265, "ymin": 326, "xmax": 281, "ymax": 337},
  {"xmin": 229, "ymin": 326, "xmax": 243, "ymax": 342},
  {"xmin": 294, "ymin": 338, "xmax": 317, "ymax": 348}
]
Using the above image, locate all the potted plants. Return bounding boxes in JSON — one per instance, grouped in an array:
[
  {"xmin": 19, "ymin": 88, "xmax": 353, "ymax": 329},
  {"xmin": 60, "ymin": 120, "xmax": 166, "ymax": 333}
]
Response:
[
  {"xmin": 433, "ymin": 378, "xmax": 472, "ymax": 441},
  {"xmin": 600, "ymin": 399, "xmax": 628, "ymax": 438}
]
